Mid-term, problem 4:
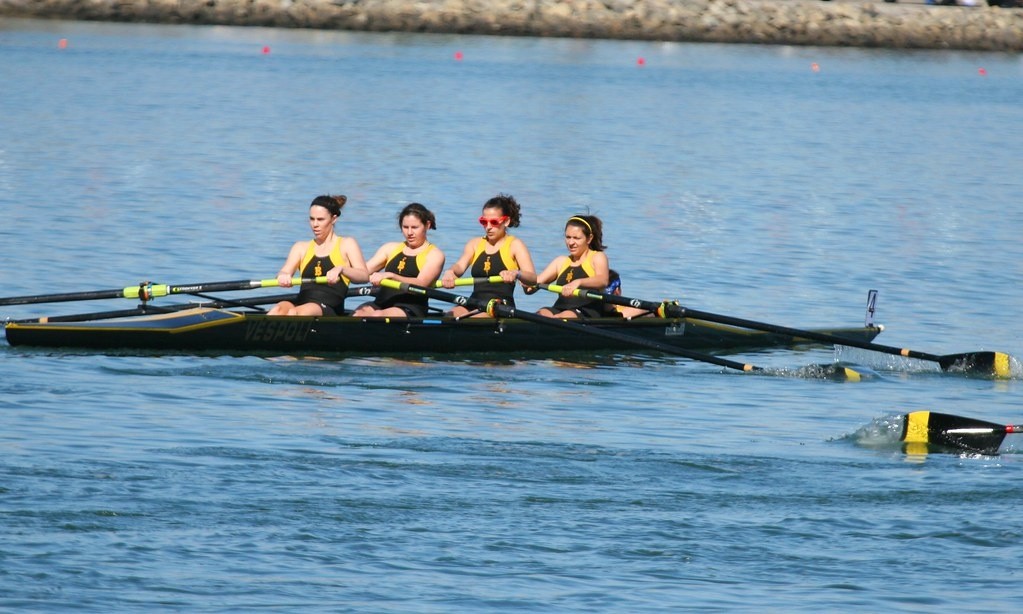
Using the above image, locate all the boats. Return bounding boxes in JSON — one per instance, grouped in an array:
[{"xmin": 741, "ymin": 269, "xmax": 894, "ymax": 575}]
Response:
[{"xmin": 3, "ymin": 267, "xmax": 884, "ymax": 362}]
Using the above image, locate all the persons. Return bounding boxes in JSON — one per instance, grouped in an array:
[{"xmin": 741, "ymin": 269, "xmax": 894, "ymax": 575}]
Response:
[
  {"xmin": 352, "ymin": 203, "xmax": 446, "ymax": 322},
  {"xmin": 519, "ymin": 211, "xmax": 610, "ymax": 319},
  {"xmin": 598, "ymin": 266, "xmax": 670, "ymax": 321},
  {"xmin": 265, "ymin": 193, "xmax": 370, "ymax": 317},
  {"xmin": 441, "ymin": 193, "xmax": 537, "ymax": 319}
]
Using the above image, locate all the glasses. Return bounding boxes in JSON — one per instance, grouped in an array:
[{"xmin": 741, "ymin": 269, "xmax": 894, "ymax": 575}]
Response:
[{"xmin": 479, "ymin": 216, "xmax": 508, "ymax": 227}]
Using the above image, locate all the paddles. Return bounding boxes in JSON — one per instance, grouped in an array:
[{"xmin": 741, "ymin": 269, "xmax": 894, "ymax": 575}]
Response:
[
  {"xmin": 374, "ymin": 279, "xmax": 1022, "ymax": 380},
  {"xmin": 0, "ymin": 275, "xmax": 513, "ymax": 328},
  {"xmin": 898, "ymin": 411, "xmax": 1023, "ymax": 457}
]
